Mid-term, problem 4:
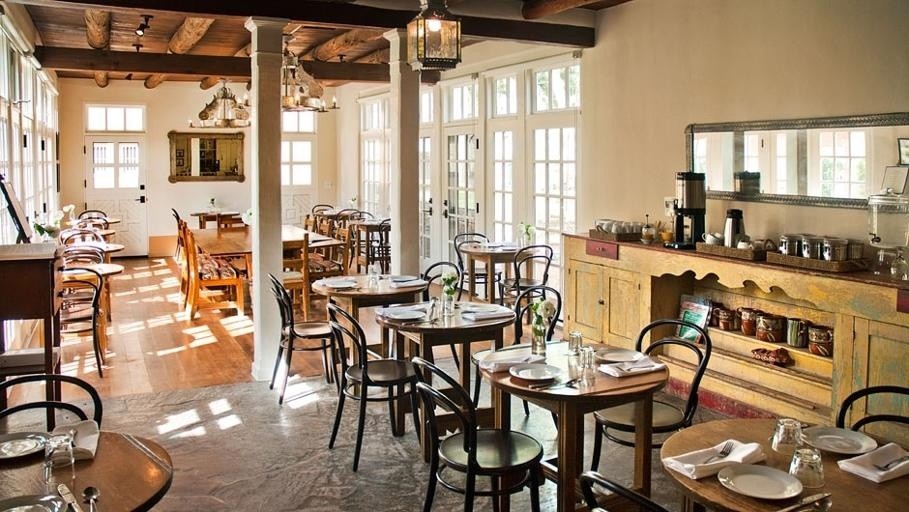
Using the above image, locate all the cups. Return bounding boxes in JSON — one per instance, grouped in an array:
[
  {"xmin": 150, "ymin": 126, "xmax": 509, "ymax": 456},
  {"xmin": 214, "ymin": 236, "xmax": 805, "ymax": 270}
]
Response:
[
  {"xmin": 577, "ymin": 343, "xmax": 596, "ymax": 378},
  {"xmin": 711, "ymin": 305, "xmax": 834, "ymax": 357},
  {"xmin": 367, "ymin": 265, "xmax": 379, "ymax": 289},
  {"xmin": 778, "ymin": 231, "xmax": 864, "ymax": 261},
  {"xmin": 480, "ymin": 238, "xmax": 488, "ymax": 251},
  {"xmin": 661, "ymin": 232, "xmax": 673, "ymax": 242},
  {"xmin": 568, "ymin": 357, "xmax": 583, "ymax": 377},
  {"xmin": 772, "ymin": 417, "xmax": 804, "ymax": 456},
  {"xmin": 699, "ymin": 231, "xmax": 775, "ymax": 251},
  {"xmin": 787, "ymin": 446, "xmax": 826, "ymax": 489},
  {"xmin": 596, "ymin": 219, "xmax": 641, "ymax": 234},
  {"xmin": 568, "ymin": 331, "xmax": 582, "ymax": 356},
  {"xmin": 428, "ymin": 296, "xmax": 440, "ymax": 321},
  {"xmin": 578, "ymin": 374, "xmax": 596, "ymax": 393},
  {"xmin": 43, "ymin": 434, "xmax": 75, "ymax": 485}
]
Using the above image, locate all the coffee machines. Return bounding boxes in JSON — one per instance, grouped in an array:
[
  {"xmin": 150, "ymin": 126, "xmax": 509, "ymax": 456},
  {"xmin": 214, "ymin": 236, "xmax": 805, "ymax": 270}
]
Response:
[
  {"xmin": 733, "ymin": 170, "xmax": 760, "ymax": 193},
  {"xmin": 664, "ymin": 171, "xmax": 707, "ymax": 249}
]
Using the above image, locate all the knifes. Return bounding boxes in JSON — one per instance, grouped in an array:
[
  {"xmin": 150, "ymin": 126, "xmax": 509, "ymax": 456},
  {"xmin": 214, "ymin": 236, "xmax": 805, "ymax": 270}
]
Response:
[
  {"xmin": 57, "ymin": 484, "xmax": 83, "ymax": 511},
  {"xmin": 775, "ymin": 491, "xmax": 832, "ymax": 511}
]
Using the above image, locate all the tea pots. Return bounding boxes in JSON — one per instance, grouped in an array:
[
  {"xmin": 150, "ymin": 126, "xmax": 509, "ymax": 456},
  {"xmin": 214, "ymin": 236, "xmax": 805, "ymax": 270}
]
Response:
[{"xmin": 723, "ymin": 208, "xmax": 745, "ymax": 247}]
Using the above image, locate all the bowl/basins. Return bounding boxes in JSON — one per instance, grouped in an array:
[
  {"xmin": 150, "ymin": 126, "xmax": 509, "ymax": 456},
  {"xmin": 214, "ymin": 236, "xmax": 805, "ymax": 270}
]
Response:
[{"xmin": 640, "ymin": 238, "xmax": 653, "ymax": 245}]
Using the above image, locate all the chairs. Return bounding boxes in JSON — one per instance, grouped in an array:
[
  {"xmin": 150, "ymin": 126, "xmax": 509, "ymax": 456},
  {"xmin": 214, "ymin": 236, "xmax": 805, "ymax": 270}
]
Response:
[
  {"xmin": 835, "ymin": 386, "xmax": 909, "ymax": 431},
  {"xmin": 172, "ymin": 208, "xmax": 245, "ymax": 320},
  {"xmin": 57, "ymin": 208, "xmax": 109, "ymax": 378},
  {"xmin": 302, "ymin": 205, "xmax": 391, "ymax": 275},
  {"xmin": 1, "ymin": 375, "xmax": 103, "ymax": 431},
  {"xmin": 268, "ymin": 233, "xmax": 711, "ymax": 512}
]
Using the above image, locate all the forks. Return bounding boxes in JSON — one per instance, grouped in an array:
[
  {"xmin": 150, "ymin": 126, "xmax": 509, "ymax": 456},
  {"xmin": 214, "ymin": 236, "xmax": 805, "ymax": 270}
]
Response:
[
  {"xmin": 700, "ymin": 440, "xmax": 734, "ymax": 464},
  {"xmin": 615, "ymin": 365, "xmax": 655, "ymax": 371},
  {"xmin": 873, "ymin": 455, "xmax": 909, "ymax": 471}
]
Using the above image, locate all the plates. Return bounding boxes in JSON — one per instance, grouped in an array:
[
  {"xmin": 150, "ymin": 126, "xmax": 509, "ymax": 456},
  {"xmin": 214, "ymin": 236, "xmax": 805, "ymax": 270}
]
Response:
[
  {"xmin": 719, "ymin": 464, "xmax": 804, "ymax": 500},
  {"xmin": 502, "ymin": 246, "xmax": 517, "ymax": 251},
  {"xmin": 0, "ymin": 432, "xmax": 50, "ymax": 460},
  {"xmin": 386, "ymin": 311, "xmax": 426, "ymax": 320},
  {"xmin": 509, "ymin": 363, "xmax": 562, "ymax": 380},
  {"xmin": 0, "ymin": 493, "xmax": 69, "ymax": 511},
  {"xmin": 594, "ymin": 348, "xmax": 644, "ymax": 362},
  {"xmin": 325, "ymin": 280, "xmax": 358, "ymax": 288},
  {"xmin": 800, "ymin": 425, "xmax": 878, "ymax": 454},
  {"xmin": 461, "ymin": 304, "xmax": 497, "ymax": 313},
  {"xmin": 390, "ymin": 275, "xmax": 419, "ymax": 282}
]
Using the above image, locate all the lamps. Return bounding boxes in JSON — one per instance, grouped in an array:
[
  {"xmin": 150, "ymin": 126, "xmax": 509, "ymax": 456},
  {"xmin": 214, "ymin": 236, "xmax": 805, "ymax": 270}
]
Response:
[
  {"xmin": 281, "ymin": 32, "xmax": 340, "ymax": 115},
  {"xmin": 403, "ymin": 0, "xmax": 466, "ymax": 84},
  {"xmin": 135, "ymin": 13, "xmax": 156, "ymax": 37},
  {"xmin": 185, "ymin": 78, "xmax": 252, "ymax": 131}
]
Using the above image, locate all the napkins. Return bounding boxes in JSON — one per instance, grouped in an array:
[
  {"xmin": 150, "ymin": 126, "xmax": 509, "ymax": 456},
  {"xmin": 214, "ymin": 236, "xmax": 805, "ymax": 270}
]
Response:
[
  {"xmin": 54, "ymin": 419, "xmax": 100, "ymax": 460},
  {"xmin": 663, "ymin": 438, "xmax": 768, "ymax": 481},
  {"xmin": 837, "ymin": 441, "xmax": 909, "ymax": 484}
]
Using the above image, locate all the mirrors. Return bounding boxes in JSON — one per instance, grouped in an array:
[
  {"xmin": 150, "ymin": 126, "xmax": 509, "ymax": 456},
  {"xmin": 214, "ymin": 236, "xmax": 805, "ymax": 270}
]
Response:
[
  {"xmin": 165, "ymin": 128, "xmax": 248, "ymax": 185},
  {"xmin": 684, "ymin": 111, "xmax": 908, "ymax": 214}
]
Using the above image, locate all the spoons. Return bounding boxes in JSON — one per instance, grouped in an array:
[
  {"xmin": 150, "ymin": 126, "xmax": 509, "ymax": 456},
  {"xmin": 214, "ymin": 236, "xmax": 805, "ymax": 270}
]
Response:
[
  {"xmin": 334, "ymin": 287, "xmax": 362, "ymax": 292},
  {"xmin": 401, "ymin": 318, "xmax": 438, "ymax": 326},
  {"xmin": 801, "ymin": 497, "xmax": 833, "ymax": 512},
  {"xmin": 80, "ymin": 485, "xmax": 101, "ymax": 511},
  {"xmin": 528, "ymin": 378, "xmax": 578, "ymax": 389}
]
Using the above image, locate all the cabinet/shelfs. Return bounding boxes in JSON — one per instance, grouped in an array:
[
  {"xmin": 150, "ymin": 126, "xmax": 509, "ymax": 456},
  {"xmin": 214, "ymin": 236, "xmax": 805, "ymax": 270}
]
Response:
[
  {"xmin": 652, "ymin": 251, "xmax": 855, "ymax": 430},
  {"xmin": 189, "ymin": 136, "xmax": 219, "ymax": 176},
  {"xmin": 0, "ymin": 243, "xmax": 61, "ymax": 431},
  {"xmin": 214, "ymin": 135, "xmax": 242, "ymax": 176},
  {"xmin": 851, "ymin": 318, "xmax": 909, "ymax": 440}
]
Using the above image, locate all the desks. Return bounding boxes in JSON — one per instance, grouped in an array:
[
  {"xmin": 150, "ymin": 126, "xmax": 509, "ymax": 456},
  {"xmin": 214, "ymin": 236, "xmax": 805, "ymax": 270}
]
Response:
[
  {"xmin": 659, "ymin": 419, "xmax": 908, "ymax": 512},
  {"xmin": 1, "ymin": 430, "xmax": 173, "ymax": 511}
]
